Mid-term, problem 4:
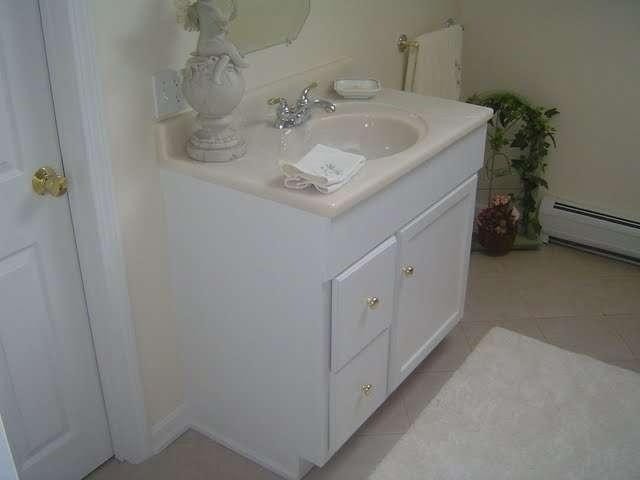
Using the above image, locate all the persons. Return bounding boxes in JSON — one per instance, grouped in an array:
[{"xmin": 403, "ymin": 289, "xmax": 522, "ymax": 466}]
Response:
[{"xmin": 191, "ymin": 1, "xmax": 250, "ymax": 87}]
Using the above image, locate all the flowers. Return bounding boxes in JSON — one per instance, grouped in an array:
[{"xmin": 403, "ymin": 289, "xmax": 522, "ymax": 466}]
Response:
[{"xmin": 478, "ymin": 191, "xmax": 520, "ymax": 233}]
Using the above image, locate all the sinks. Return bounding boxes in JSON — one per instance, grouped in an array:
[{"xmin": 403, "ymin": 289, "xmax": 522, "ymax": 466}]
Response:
[{"xmin": 286, "ymin": 102, "xmax": 428, "ymax": 161}]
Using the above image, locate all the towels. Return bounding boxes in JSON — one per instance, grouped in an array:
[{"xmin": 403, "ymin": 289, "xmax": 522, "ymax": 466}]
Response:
[
  {"xmin": 406, "ymin": 23, "xmax": 464, "ymax": 99},
  {"xmin": 283, "ymin": 140, "xmax": 369, "ymax": 193}
]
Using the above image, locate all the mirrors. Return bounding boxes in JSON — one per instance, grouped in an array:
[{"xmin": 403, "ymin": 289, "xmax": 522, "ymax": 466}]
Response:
[{"xmin": 215, "ymin": 0, "xmax": 313, "ymax": 58}]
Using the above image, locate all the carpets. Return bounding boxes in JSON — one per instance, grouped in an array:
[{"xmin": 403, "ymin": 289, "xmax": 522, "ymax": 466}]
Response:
[{"xmin": 370, "ymin": 315, "xmax": 637, "ymax": 478}]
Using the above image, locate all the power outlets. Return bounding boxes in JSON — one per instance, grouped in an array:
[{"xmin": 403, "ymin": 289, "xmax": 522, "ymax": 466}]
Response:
[{"xmin": 153, "ymin": 66, "xmax": 190, "ymax": 124}]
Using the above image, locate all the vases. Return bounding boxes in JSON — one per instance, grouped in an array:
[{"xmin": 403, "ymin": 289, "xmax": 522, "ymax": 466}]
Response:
[{"xmin": 480, "ymin": 229, "xmax": 517, "ymax": 257}]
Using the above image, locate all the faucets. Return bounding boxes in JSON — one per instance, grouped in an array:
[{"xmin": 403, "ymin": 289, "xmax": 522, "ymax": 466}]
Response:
[{"xmin": 293, "ymin": 99, "xmax": 335, "ymax": 122}]
